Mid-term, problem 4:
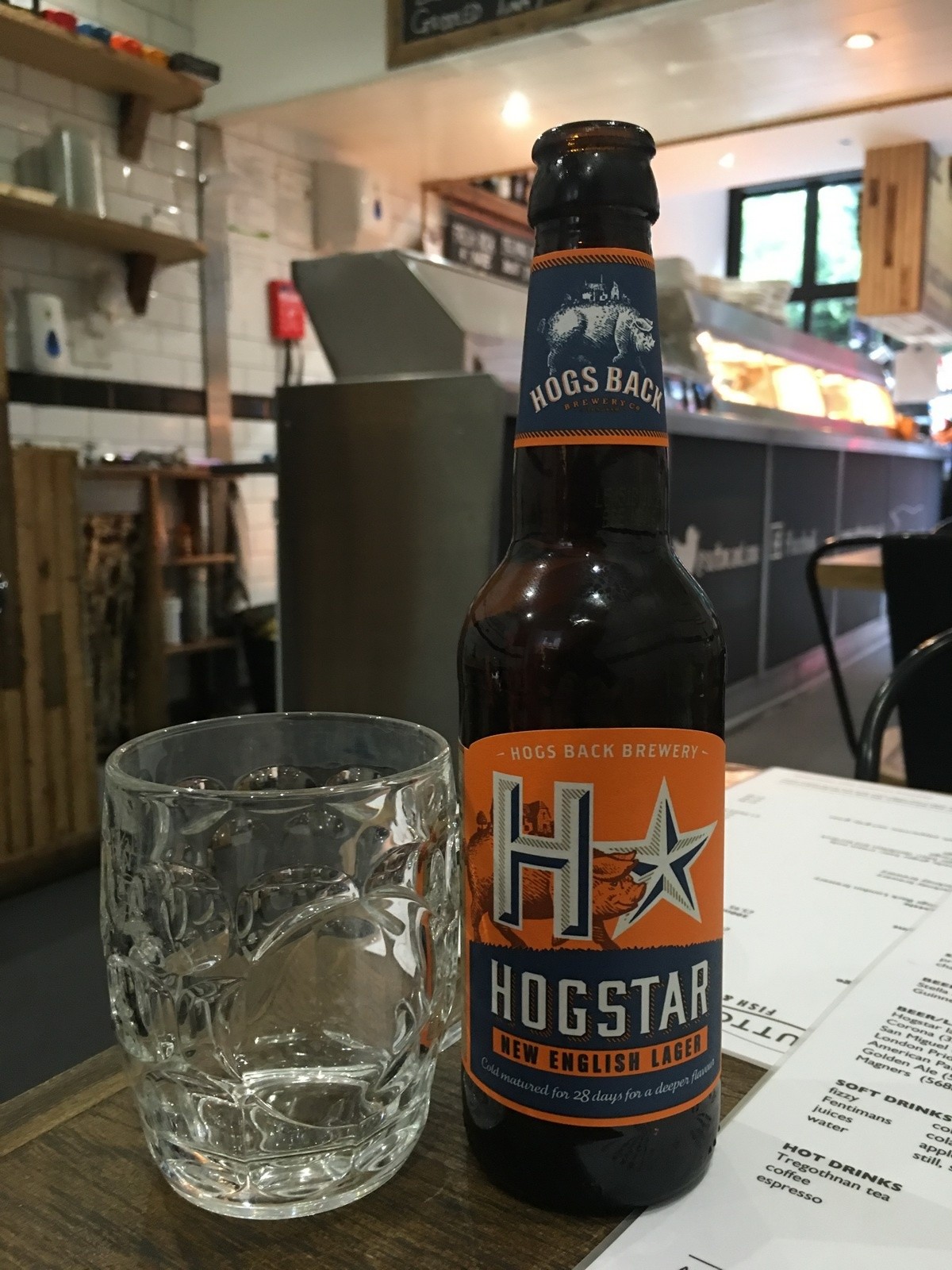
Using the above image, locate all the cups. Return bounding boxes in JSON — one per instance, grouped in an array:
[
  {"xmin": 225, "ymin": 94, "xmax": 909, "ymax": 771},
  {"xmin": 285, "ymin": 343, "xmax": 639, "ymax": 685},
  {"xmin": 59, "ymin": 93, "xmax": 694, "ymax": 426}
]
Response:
[
  {"xmin": 97, "ymin": 709, "xmax": 465, "ymax": 1219},
  {"xmin": 50, "ymin": 126, "xmax": 111, "ymax": 219},
  {"xmin": 27, "ymin": 290, "xmax": 72, "ymax": 376}
]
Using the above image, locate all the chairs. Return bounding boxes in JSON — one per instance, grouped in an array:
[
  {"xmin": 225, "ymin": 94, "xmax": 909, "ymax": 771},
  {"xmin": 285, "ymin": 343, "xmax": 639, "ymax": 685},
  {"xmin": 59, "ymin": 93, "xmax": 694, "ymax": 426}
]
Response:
[{"xmin": 807, "ymin": 521, "xmax": 952, "ymax": 793}]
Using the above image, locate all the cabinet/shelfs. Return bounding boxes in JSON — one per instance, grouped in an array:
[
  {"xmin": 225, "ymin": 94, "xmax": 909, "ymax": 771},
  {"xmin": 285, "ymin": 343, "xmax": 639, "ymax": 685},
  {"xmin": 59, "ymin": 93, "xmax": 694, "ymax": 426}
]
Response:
[{"xmin": 0, "ymin": 0, "xmax": 229, "ymax": 876}]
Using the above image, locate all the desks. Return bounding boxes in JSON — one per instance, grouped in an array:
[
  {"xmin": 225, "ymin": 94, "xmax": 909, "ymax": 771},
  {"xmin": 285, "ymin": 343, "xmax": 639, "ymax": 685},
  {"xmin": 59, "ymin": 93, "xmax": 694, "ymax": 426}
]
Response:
[{"xmin": 0, "ymin": 762, "xmax": 952, "ymax": 1270}]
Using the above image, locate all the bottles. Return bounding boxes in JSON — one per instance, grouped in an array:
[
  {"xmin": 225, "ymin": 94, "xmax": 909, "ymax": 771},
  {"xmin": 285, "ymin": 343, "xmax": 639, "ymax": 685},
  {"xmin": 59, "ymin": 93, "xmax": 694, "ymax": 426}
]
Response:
[{"xmin": 459, "ymin": 118, "xmax": 727, "ymax": 1216}]
